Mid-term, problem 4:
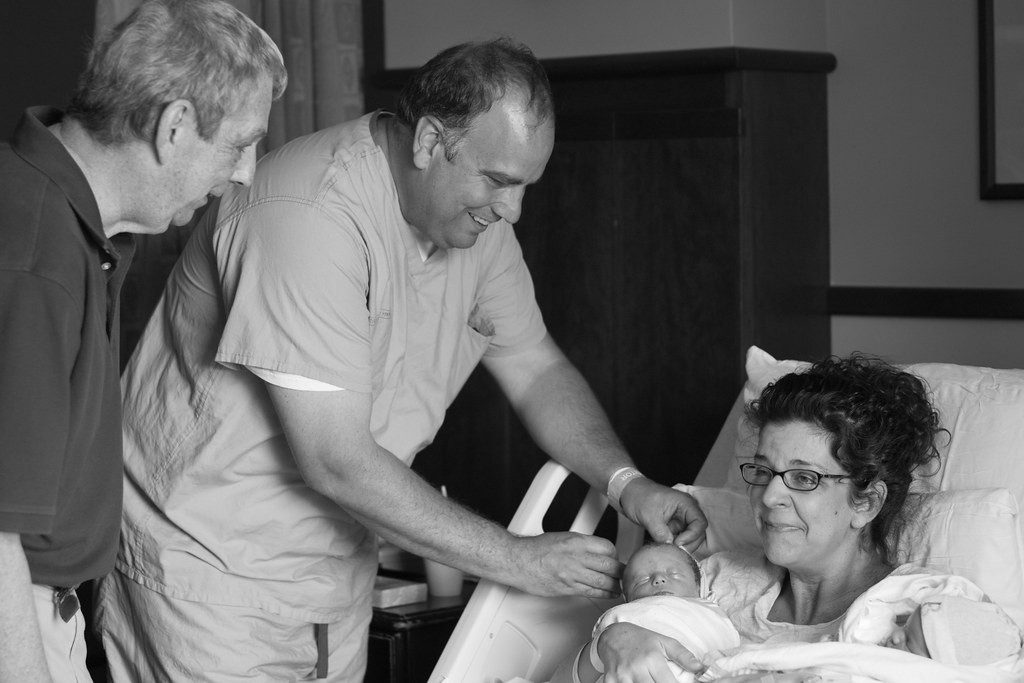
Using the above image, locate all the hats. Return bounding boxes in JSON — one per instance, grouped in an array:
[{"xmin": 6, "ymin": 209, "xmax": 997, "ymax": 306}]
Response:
[{"xmin": 919, "ymin": 594, "xmax": 1022, "ymax": 665}]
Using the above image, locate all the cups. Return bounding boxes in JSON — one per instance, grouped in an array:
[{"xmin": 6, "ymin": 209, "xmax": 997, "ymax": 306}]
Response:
[{"xmin": 424, "ymin": 558, "xmax": 465, "ymax": 597}]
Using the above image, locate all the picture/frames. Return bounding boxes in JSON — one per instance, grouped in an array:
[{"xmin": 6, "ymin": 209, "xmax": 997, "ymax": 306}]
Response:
[{"xmin": 976, "ymin": 0, "xmax": 1024, "ymax": 202}]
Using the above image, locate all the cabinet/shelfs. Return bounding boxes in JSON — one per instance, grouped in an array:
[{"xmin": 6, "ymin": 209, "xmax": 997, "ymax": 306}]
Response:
[{"xmin": 362, "ymin": 48, "xmax": 839, "ymax": 547}]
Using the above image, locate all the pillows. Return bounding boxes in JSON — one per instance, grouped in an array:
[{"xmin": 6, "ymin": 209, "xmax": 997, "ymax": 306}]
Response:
[
  {"xmin": 723, "ymin": 345, "xmax": 1024, "ymax": 541},
  {"xmin": 670, "ymin": 483, "xmax": 1024, "ymax": 644}
]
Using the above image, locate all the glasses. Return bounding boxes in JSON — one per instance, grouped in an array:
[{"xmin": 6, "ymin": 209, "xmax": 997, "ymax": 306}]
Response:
[{"xmin": 738, "ymin": 462, "xmax": 852, "ymax": 493}]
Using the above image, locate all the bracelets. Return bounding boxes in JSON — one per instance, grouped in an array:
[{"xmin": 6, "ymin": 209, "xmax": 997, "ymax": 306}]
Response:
[
  {"xmin": 588, "ymin": 625, "xmax": 604, "ymax": 675},
  {"xmin": 570, "ymin": 640, "xmax": 588, "ymax": 682},
  {"xmin": 604, "ymin": 466, "xmax": 646, "ymax": 523}
]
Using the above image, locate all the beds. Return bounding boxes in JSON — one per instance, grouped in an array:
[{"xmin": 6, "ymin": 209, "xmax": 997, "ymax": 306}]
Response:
[{"xmin": 426, "ymin": 459, "xmax": 1024, "ymax": 683}]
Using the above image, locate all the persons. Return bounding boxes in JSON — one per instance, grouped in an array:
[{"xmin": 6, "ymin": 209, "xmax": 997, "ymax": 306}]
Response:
[
  {"xmin": 86, "ymin": 39, "xmax": 709, "ymax": 683},
  {"xmin": 547, "ymin": 347, "xmax": 1024, "ymax": 683},
  {"xmin": 0, "ymin": 0, "xmax": 291, "ymax": 683}
]
